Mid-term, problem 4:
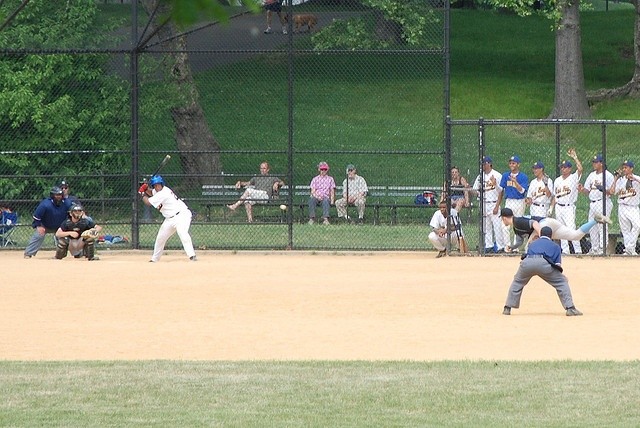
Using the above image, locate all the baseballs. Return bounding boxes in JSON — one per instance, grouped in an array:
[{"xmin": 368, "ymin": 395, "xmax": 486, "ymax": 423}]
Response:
[{"xmin": 280, "ymin": 204, "xmax": 287, "ymax": 210}]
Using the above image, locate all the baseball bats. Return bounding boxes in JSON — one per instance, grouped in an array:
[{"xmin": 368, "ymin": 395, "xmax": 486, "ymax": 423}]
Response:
[
  {"xmin": 457, "ymin": 212, "xmax": 464, "ymax": 253},
  {"xmin": 146, "ymin": 154, "xmax": 171, "ymax": 184},
  {"xmin": 450, "ymin": 215, "xmax": 460, "ymax": 246},
  {"xmin": 454, "ymin": 215, "xmax": 468, "ymax": 253}
]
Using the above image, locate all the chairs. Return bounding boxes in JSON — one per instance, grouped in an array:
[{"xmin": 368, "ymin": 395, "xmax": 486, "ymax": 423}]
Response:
[{"xmin": 0, "ymin": 213, "xmax": 19, "ymax": 248}]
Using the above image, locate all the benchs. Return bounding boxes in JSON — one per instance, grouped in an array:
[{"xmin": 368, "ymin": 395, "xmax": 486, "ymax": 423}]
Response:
[
  {"xmin": 179, "ymin": 181, "xmax": 291, "ymax": 224},
  {"xmin": 384, "ymin": 183, "xmax": 480, "ymax": 227},
  {"xmin": 291, "ymin": 185, "xmax": 391, "ymax": 226}
]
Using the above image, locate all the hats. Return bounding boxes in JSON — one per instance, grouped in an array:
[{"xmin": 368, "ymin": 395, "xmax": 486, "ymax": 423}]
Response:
[
  {"xmin": 558, "ymin": 160, "xmax": 572, "ymax": 168},
  {"xmin": 348, "ymin": 165, "xmax": 354, "ymax": 171},
  {"xmin": 592, "ymin": 155, "xmax": 603, "ymax": 162},
  {"xmin": 498, "ymin": 208, "xmax": 513, "ymax": 218},
  {"xmin": 531, "ymin": 162, "xmax": 544, "ymax": 168},
  {"xmin": 58, "ymin": 181, "xmax": 68, "ymax": 188},
  {"xmin": 509, "ymin": 156, "xmax": 521, "ymax": 164},
  {"xmin": 318, "ymin": 162, "xmax": 330, "ymax": 172},
  {"xmin": 622, "ymin": 160, "xmax": 634, "ymax": 167},
  {"xmin": 480, "ymin": 156, "xmax": 492, "ymax": 163},
  {"xmin": 541, "ymin": 226, "xmax": 552, "ymax": 236}
]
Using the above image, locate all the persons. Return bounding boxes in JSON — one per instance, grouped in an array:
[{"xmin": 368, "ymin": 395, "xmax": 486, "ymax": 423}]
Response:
[
  {"xmin": 24, "ymin": 186, "xmax": 73, "ymax": 259},
  {"xmin": 502, "ymin": 226, "xmax": 583, "ymax": 316},
  {"xmin": 307, "ymin": 161, "xmax": 336, "ymax": 225},
  {"xmin": 577, "ymin": 155, "xmax": 615, "ymax": 254},
  {"xmin": 58, "ymin": 180, "xmax": 81, "ymax": 206},
  {"xmin": 493, "ymin": 155, "xmax": 528, "ymax": 253},
  {"xmin": 546, "ymin": 148, "xmax": 582, "ymax": 255},
  {"xmin": 335, "ymin": 164, "xmax": 369, "ymax": 225},
  {"xmin": 498, "ymin": 206, "xmax": 613, "ymax": 254},
  {"xmin": 54, "ymin": 205, "xmax": 103, "ymax": 261},
  {"xmin": 427, "ymin": 201, "xmax": 464, "ymax": 258},
  {"xmin": 609, "ymin": 159, "xmax": 640, "ymax": 254},
  {"xmin": 526, "ymin": 162, "xmax": 553, "ymax": 217},
  {"xmin": 141, "ymin": 174, "xmax": 197, "ymax": 262},
  {"xmin": 465, "ymin": 156, "xmax": 504, "ymax": 253},
  {"xmin": 438, "ymin": 165, "xmax": 470, "ymax": 213},
  {"xmin": 0, "ymin": 203, "xmax": 17, "ymax": 235},
  {"xmin": 226, "ymin": 162, "xmax": 284, "ymax": 222}
]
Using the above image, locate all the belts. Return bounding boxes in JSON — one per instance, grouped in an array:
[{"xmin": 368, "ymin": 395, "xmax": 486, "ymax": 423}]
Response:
[
  {"xmin": 558, "ymin": 203, "xmax": 570, "ymax": 206},
  {"xmin": 532, "ymin": 202, "xmax": 545, "ymax": 207},
  {"xmin": 486, "ymin": 201, "xmax": 497, "ymax": 203},
  {"xmin": 170, "ymin": 212, "xmax": 179, "ymax": 218}
]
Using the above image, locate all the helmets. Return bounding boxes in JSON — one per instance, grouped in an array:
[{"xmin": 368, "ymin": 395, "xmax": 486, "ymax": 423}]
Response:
[
  {"xmin": 149, "ymin": 174, "xmax": 165, "ymax": 189},
  {"xmin": 50, "ymin": 187, "xmax": 65, "ymax": 207},
  {"xmin": 67, "ymin": 203, "xmax": 85, "ymax": 219}
]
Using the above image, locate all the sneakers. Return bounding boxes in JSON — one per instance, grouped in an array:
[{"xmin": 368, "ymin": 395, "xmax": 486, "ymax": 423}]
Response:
[
  {"xmin": 348, "ymin": 218, "xmax": 353, "ymax": 225},
  {"xmin": 323, "ymin": 219, "xmax": 330, "ymax": 225},
  {"xmin": 88, "ymin": 256, "xmax": 99, "ymax": 260},
  {"xmin": 503, "ymin": 306, "xmax": 511, "ymax": 315},
  {"xmin": 593, "ymin": 211, "xmax": 613, "ymax": 225},
  {"xmin": 190, "ymin": 255, "xmax": 198, "ymax": 261},
  {"xmin": 435, "ymin": 247, "xmax": 446, "ymax": 258},
  {"xmin": 566, "ymin": 307, "xmax": 583, "ymax": 316},
  {"xmin": 24, "ymin": 251, "xmax": 32, "ymax": 259},
  {"xmin": 308, "ymin": 218, "xmax": 314, "ymax": 225},
  {"xmin": 356, "ymin": 218, "xmax": 364, "ymax": 224}
]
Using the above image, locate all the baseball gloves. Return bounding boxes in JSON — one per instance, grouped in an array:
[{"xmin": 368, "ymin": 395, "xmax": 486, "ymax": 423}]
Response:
[{"xmin": 81, "ymin": 228, "xmax": 98, "ymax": 238}]
